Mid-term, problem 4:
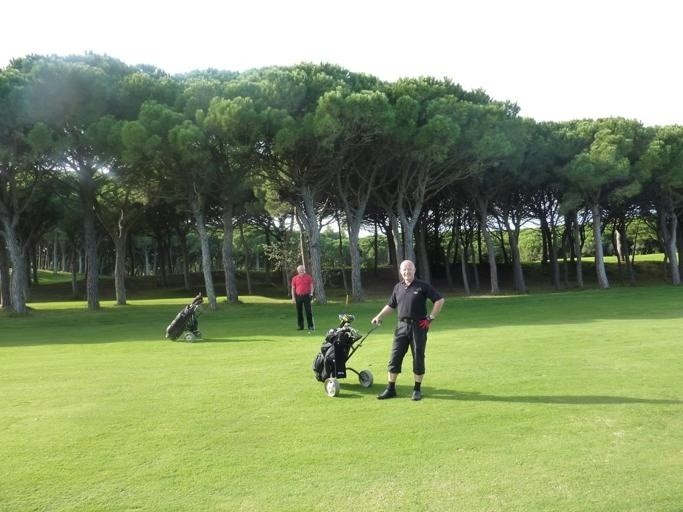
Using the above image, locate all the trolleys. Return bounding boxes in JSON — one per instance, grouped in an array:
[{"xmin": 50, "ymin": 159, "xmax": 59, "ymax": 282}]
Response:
[
  {"xmin": 166, "ymin": 295, "xmax": 216, "ymax": 342},
  {"xmin": 314, "ymin": 314, "xmax": 381, "ymax": 397}
]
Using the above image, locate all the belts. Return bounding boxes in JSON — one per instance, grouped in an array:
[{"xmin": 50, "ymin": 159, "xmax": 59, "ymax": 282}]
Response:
[{"xmin": 402, "ymin": 318, "xmax": 420, "ymax": 325}]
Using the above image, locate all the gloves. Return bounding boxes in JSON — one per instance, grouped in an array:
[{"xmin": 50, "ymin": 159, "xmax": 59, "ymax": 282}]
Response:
[{"xmin": 418, "ymin": 315, "xmax": 434, "ymax": 329}]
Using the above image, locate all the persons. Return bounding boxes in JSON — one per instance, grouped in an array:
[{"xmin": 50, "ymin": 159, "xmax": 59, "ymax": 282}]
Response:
[
  {"xmin": 369, "ymin": 260, "xmax": 445, "ymax": 402},
  {"xmin": 290, "ymin": 265, "xmax": 316, "ymax": 331}
]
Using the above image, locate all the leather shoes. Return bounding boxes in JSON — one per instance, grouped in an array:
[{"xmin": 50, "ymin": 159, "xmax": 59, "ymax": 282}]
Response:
[
  {"xmin": 412, "ymin": 390, "xmax": 421, "ymax": 400},
  {"xmin": 376, "ymin": 389, "xmax": 396, "ymax": 400}
]
